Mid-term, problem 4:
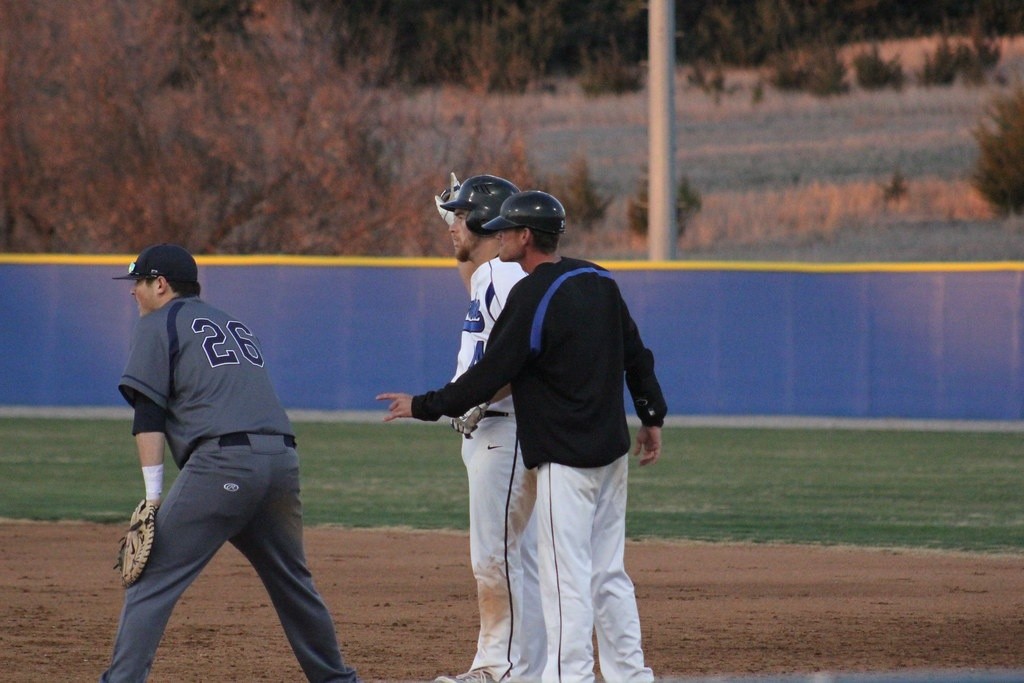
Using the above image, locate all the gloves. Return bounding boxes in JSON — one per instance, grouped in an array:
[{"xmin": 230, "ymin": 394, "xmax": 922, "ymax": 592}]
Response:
[{"xmin": 450, "ymin": 402, "xmax": 489, "ymax": 439}]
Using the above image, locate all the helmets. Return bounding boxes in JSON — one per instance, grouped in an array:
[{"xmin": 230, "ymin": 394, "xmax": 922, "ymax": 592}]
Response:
[
  {"xmin": 440, "ymin": 175, "xmax": 522, "ymax": 234},
  {"xmin": 482, "ymin": 190, "xmax": 566, "ymax": 233}
]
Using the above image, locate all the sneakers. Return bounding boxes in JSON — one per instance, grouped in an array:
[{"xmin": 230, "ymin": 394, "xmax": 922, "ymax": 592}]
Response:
[{"xmin": 434, "ymin": 668, "xmax": 498, "ymax": 683}]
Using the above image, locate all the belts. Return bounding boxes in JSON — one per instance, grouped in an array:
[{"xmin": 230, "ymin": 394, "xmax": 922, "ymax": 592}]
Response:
[
  {"xmin": 219, "ymin": 432, "xmax": 296, "ymax": 448},
  {"xmin": 483, "ymin": 409, "xmax": 508, "ymax": 417}
]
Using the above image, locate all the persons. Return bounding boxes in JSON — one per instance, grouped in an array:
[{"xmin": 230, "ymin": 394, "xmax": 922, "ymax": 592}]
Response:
[
  {"xmin": 100, "ymin": 242, "xmax": 356, "ymax": 683},
  {"xmin": 435, "ymin": 171, "xmax": 546, "ymax": 683},
  {"xmin": 375, "ymin": 189, "xmax": 667, "ymax": 682}
]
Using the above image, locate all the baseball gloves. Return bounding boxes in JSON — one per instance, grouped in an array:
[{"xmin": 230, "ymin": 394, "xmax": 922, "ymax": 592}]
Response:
[{"xmin": 118, "ymin": 498, "xmax": 158, "ymax": 589}]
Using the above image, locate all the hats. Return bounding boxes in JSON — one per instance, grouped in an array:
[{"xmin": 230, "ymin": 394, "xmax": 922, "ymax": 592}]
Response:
[{"xmin": 112, "ymin": 242, "xmax": 197, "ymax": 282}]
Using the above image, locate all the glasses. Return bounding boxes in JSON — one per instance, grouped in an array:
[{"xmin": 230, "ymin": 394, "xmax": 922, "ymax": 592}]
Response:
[{"xmin": 128, "ymin": 261, "xmax": 157, "ymax": 275}]
